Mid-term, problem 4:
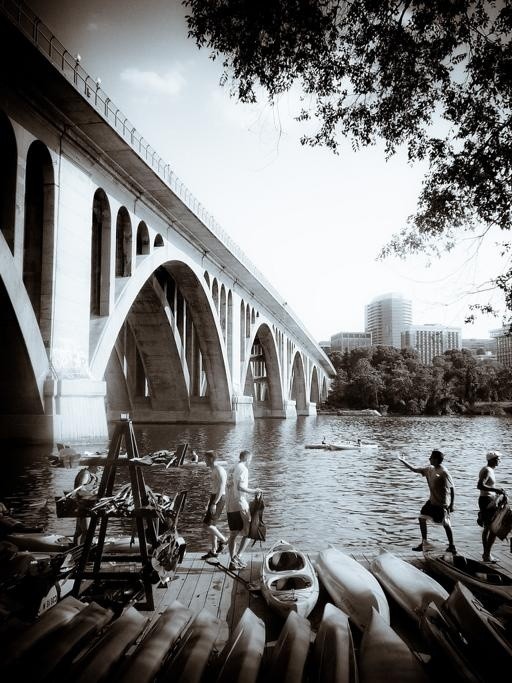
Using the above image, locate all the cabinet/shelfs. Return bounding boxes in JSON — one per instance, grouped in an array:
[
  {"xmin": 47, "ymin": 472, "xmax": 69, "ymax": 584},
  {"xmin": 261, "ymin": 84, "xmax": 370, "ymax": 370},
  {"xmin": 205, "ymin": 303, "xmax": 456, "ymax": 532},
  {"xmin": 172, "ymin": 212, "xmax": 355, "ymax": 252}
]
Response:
[{"xmin": 60, "ymin": 418, "xmax": 164, "ymax": 611}]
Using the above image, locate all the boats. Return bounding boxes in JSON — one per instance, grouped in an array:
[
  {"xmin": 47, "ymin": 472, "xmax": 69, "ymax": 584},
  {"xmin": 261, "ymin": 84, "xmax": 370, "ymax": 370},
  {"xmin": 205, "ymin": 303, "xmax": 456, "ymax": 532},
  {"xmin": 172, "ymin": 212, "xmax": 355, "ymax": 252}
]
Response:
[
  {"xmin": 259, "ymin": 537, "xmax": 321, "ymax": 619},
  {"xmin": 7, "ymin": 531, "xmax": 153, "ymax": 552},
  {"xmin": 422, "ymin": 549, "xmax": 512, "ymax": 605},
  {"xmin": 180, "ymin": 461, "xmax": 228, "ymax": 468},
  {"xmin": 304, "ymin": 443, "xmax": 359, "ymax": 451},
  {"xmin": 368, "ymin": 545, "xmax": 451, "ymax": 621},
  {"xmin": 313, "ymin": 542, "xmax": 394, "ymax": 632}
]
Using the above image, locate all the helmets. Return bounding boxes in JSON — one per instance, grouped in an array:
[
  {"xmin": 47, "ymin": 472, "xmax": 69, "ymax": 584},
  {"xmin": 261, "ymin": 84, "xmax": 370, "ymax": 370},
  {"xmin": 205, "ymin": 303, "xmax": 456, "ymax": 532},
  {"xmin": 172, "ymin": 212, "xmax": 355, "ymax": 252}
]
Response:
[{"xmin": 486, "ymin": 450, "xmax": 503, "ymax": 460}]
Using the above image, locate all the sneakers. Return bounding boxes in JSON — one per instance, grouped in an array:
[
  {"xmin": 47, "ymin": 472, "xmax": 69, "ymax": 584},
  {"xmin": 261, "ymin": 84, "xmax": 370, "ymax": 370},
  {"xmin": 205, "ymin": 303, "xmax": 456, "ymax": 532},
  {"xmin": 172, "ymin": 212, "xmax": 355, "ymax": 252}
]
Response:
[
  {"xmin": 229, "ymin": 562, "xmax": 242, "ymax": 570},
  {"xmin": 476, "ymin": 512, "xmax": 484, "ymax": 527},
  {"xmin": 412, "ymin": 542, "xmax": 423, "ymax": 551},
  {"xmin": 445, "ymin": 545, "xmax": 458, "ymax": 555},
  {"xmin": 202, "ymin": 551, "xmax": 218, "ymax": 559},
  {"xmin": 216, "ymin": 538, "xmax": 229, "ymax": 553},
  {"xmin": 232, "ymin": 555, "xmax": 248, "ymax": 568}
]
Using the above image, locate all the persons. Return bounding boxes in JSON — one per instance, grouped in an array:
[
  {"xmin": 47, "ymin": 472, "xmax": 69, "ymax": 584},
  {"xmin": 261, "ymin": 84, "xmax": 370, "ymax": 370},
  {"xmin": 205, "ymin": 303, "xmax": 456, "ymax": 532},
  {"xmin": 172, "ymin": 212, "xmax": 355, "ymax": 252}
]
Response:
[
  {"xmin": 477, "ymin": 451, "xmax": 507, "ymax": 564},
  {"xmin": 399, "ymin": 449, "xmax": 454, "ymax": 553},
  {"xmin": 73, "ymin": 465, "xmax": 101, "ymax": 546},
  {"xmin": 225, "ymin": 451, "xmax": 262, "ymax": 571},
  {"xmin": 201, "ymin": 449, "xmax": 228, "ymax": 558},
  {"xmin": 189, "ymin": 451, "xmax": 199, "ymax": 463}
]
